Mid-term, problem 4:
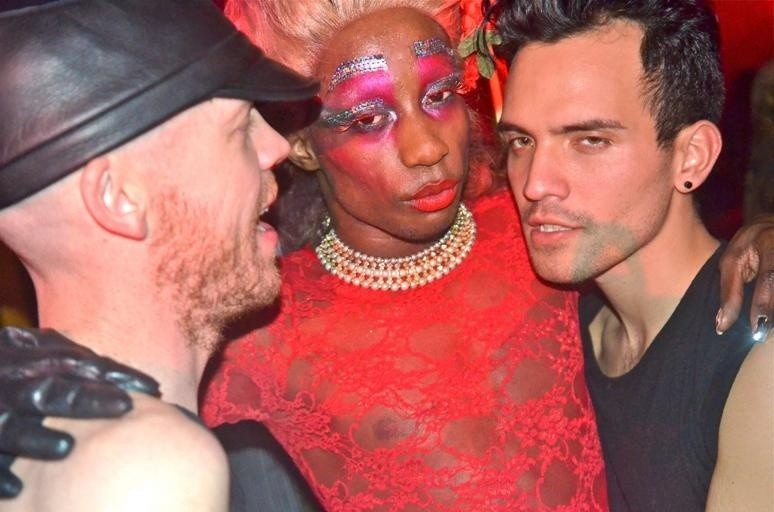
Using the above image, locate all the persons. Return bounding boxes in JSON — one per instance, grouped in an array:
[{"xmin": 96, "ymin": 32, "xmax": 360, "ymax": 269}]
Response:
[
  {"xmin": 471, "ymin": 0, "xmax": 774, "ymax": 511},
  {"xmin": 1, "ymin": 2, "xmax": 774, "ymax": 511},
  {"xmin": 1, "ymin": 1, "xmax": 320, "ymax": 512}
]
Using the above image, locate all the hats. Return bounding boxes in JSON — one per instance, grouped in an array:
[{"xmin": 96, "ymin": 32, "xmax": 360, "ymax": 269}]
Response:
[{"xmin": 0, "ymin": 2, "xmax": 322, "ymax": 212}]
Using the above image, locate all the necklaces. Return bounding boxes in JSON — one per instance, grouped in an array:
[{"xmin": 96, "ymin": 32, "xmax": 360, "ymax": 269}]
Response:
[{"xmin": 312, "ymin": 200, "xmax": 478, "ymax": 292}]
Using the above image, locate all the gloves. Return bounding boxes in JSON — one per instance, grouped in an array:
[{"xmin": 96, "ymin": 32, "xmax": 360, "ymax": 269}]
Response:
[{"xmin": 0, "ymin": 324, "xmax": 161, "ymax": 503}]
